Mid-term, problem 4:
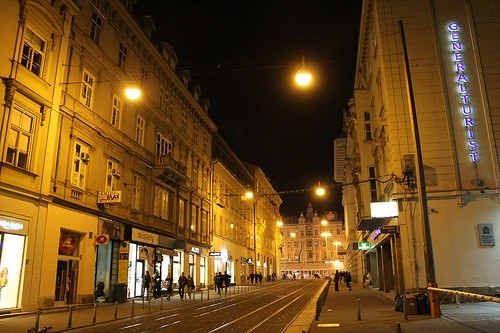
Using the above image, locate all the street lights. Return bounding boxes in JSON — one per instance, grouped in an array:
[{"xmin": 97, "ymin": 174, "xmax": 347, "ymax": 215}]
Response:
[
  {"xmin": 321, "ymin": 232, "xmax": 331, "ymax": 274},
  {"xmin": 333, "ymin": 242, "xmax": 341, "ymax": 260}
]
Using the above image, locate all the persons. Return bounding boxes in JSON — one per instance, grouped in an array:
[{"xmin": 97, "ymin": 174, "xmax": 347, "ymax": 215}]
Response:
[
  {"xmin": 153, "ymin": 272, "xmax": 161, "ymax": 300},
  {"xmin": 166, "ymin": 273, "xmax": 173, "ymax": 300},
  {"xmin": 250, "ymin": 271, "xmax": 262, "ymax": 285},
  {"xmin": 144, "ymin": 271, "xmax": 151, "ymax": 301},
  {"xmin": 178, "ymin": 272, "xmax": 194, "ymax": 301},
  {"xmin": 272, "ymin": 272, "xmax": 276, "ymax": 281},
  {"xmin": 294, "ymin": 274, "xmax": 295, "ymax": 280},
  {"xmin": 363, "ymin": 271, "xmax": 371, "ymax": 288},
  {"xmin": 315, "ymin": 274, "xmax": 316, "ymax": 279},
  {"xmin": 335, "ymin": 270, "xmax": 352, "ymax": 291},
  {"xmin": 214, "ymin": 271, "xmax": 231, "ymax": 295},
  {"xmin": 284, "ymin": 274, "xmax": 285, "ymax": 279}
]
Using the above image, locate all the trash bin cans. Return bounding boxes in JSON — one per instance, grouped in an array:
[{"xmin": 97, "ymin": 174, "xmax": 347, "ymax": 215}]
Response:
[{"xmin": 113, "ymin": 283, "xmax": 128, "ymax": 303}]
